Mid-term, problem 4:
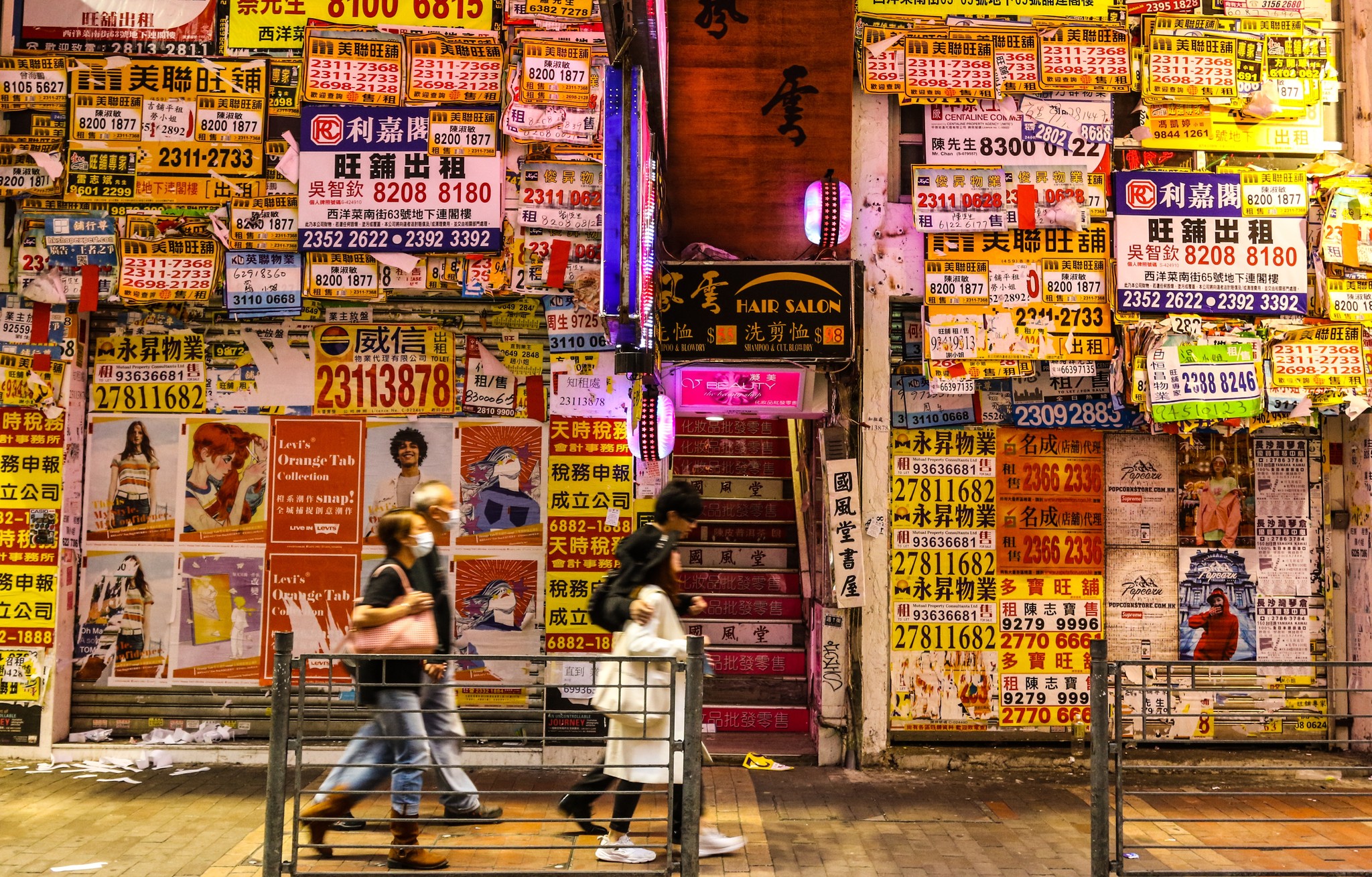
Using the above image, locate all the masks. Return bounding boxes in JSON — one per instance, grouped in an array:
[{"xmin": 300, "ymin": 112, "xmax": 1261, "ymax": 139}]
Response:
[
  {"xmin": 405, "ymin": 531, "xmax": 434, "ymax": 559},
  {"xmin": 433, "ymin": 506, "xmax": 461, "ymax": 531}
]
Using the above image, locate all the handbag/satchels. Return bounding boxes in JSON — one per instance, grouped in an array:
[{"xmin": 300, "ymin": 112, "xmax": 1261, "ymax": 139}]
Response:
[
  {"xmin": 354, "ymin": 563, "xmax": 442, "ymax": 658},
  {"xmin": 587, "ymin": 587, "xmax": 684, "ymax": 729},
  {"xmin": 588, "ymin": 567, "xmax": 629, "ymax": 634}
]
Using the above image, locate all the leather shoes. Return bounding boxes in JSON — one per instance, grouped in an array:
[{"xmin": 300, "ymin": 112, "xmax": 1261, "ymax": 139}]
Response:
[
  {"xmin": 558, "ymin": 793, "xmax": 607, "ymax": 835},
  {"xmin": 672, "ymin": 827, "xmax": 682, "ymax": 844}
]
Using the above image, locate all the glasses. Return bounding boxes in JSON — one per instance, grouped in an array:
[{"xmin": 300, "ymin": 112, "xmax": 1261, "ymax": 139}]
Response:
[
  {"xmin": 1212, "ymin": 461, "xmax": 1225, "ymax": 467},
  {"xmin": 675, "ymin": 514, "xmax": 697, "ymax": 524}
]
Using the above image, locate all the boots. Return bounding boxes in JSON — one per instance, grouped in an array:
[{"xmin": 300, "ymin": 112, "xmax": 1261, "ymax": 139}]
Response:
[
  {"xmin": 387, "ymin": 807, "xmax": 449, "ymax": 869},
  {"xmin": 298, "ymin": 785, "xmax": 360, "ymax": 857}
]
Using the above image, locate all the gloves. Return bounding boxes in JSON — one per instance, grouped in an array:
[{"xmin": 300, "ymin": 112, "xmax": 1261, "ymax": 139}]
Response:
[{"xmin": 1208, "ymin": 604, "xmax": 1223, "ymax": 616}]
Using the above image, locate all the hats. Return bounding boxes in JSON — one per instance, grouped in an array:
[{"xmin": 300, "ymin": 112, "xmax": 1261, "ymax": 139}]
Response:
[{"xmin": 619, "ymin": 523, "xmax": 680, "ymax": 570}]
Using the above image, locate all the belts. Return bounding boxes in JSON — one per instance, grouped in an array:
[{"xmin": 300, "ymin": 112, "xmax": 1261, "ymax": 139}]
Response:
[
  {"xmin": 118, "ymin": 628, "xmax": 142, "ymax": 636},
  {"xmin": 115, "ymin": 490, "xmax": 148, "ymax": 500}
]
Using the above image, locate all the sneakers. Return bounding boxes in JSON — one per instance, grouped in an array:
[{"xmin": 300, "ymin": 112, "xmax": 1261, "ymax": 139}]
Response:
[
  {"xmin": 443, "ymin": 801, "xmax": 503, "ymax": 822},
  {"xmin": 595, "ymin": 834, "xmax": 656, "ymax": 864},
  {"xmin": 696, "ymin": 828, "xmax": 746, "ymax": 858},
  {"xmin": 330, "ymin": 812, "xmax": 366, "ymax": 830}
]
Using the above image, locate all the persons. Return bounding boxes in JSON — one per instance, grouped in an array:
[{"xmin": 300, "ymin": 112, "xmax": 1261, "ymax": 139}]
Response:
[
  {"xmin": 594, "ymin": 524, "xmax": 747, "ymax": 862},
  {"xmin": 556, "ymin": 479, "xmax": 708, "ymax": 841},
  {"xmin": 364, "ymin": 427, "xmax": 431, "ymax": 535},
  {"xmin": 1188, "ymin": 591, "xmax": 1239, "ymax": 661},
  {"xmin": 300, "ymin": 509, "xmax": 450, "ymax": 870},
  {"xmin": 105, "ymin": 420, "xmax": 161, "ymax": 529},
  {"xmin": 311, "ymin": 479, "xmax": 502, "ymax": 831},
  {"xmin": 115, "ymin": 555, "xmax": 154, "ymax": 662},
  {"xmin": 1197, "ymin": 455, "xmax": 1242, "ymax": 548}
]
